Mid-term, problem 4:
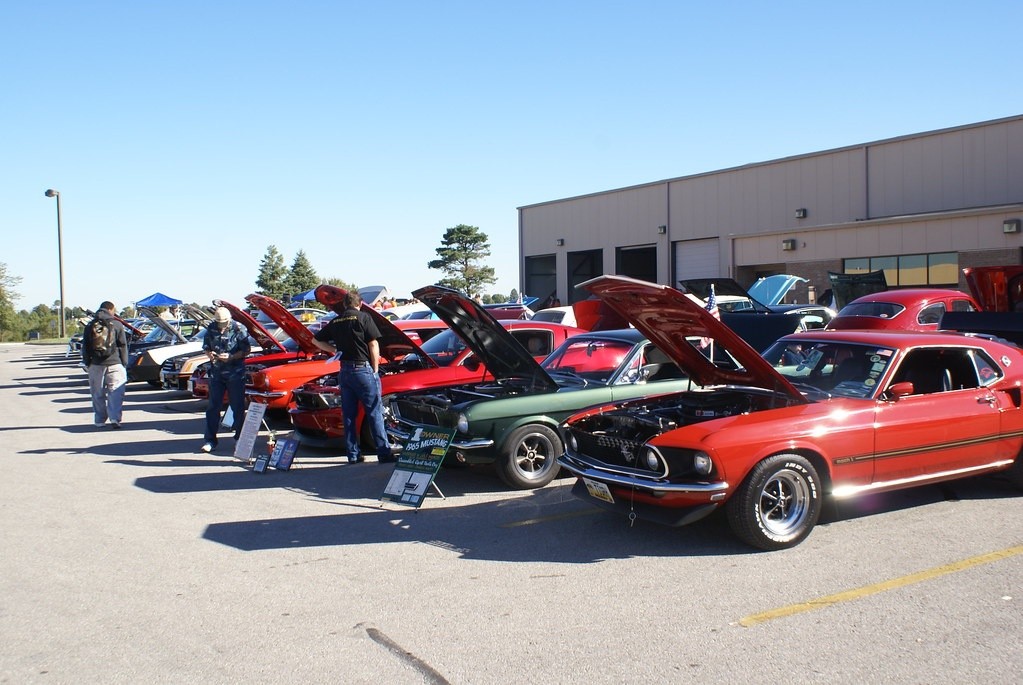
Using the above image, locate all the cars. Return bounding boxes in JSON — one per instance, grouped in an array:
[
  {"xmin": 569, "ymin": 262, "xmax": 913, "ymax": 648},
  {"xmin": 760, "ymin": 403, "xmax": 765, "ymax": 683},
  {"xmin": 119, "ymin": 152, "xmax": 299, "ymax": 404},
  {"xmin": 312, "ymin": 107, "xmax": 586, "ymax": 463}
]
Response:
[
  {"xmin": 382, "ymin": 285, "xmax": 741, "ymax": 489},
  {"xmin": 65, "ymin": 271, "xmax": 985, "ymax": 434},
  {"xmin": 553, "ymin": 272, "xmax": 1022, "ymax": 553}
]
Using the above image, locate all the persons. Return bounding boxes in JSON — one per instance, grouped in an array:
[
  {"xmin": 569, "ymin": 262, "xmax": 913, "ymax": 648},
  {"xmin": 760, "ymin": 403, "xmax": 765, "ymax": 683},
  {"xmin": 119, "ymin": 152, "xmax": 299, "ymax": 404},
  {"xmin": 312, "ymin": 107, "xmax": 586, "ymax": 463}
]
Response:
[
  {"xmin": 548, "ymin": 295, "xmax": 561, "ymax": 307},
  {"xmin": 784, "ymin": 344, "xmax": 803, "ymax": 366},
  {"xmin": 82, "ymin": 301, "xmax": 128, "ymax": 429},
  {"xmin": 471, "ymin": 293, "xmax": 484, "ymax": 304},
  {"xmin": 373, "ymin": 296, "xmax": 421, "ymax": 310},
  {"xmin": 201, "ymin": 306, "xmax": 251, "ymax": 452},
  {"xmin": 312, "ymin": 290, "xmax": 399, "ymax": 464}
]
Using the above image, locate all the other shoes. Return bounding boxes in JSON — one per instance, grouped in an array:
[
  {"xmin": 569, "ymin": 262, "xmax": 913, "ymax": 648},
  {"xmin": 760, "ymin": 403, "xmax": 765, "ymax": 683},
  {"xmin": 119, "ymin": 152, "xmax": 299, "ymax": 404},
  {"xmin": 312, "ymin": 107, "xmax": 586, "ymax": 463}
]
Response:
[
  {"xmin": 379, "ymin": 455, "xmax": 399, "ymax": 463},
  {"xmin": 348, "ymin": 457, "xmax": 365, "ymax": 464}
]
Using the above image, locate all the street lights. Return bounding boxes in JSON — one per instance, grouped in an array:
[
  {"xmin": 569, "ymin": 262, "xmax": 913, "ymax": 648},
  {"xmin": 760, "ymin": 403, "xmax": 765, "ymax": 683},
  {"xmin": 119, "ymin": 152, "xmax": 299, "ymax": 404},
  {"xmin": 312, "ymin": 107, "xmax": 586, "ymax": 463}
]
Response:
[{"xmin": 45, "ymin": 188, "xmax": 66, "ymax": 340}]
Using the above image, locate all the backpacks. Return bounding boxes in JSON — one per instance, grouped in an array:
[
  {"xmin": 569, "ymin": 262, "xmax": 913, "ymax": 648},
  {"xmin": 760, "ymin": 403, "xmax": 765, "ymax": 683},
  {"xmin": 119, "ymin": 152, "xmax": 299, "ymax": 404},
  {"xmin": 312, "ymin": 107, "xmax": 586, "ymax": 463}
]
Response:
[{"xmin": 89, "ymin": 318, "xmax": 116, "ymax": 363}]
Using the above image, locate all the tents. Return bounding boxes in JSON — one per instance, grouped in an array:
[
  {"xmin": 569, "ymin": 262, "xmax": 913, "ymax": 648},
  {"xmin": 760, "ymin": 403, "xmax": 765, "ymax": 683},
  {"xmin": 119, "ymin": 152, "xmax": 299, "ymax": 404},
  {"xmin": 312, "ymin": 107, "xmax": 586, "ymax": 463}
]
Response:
[
  {"xmin": 291, "ymin": 288, "xmax": 319, "ymax": 308},
  {"xmin": 134, "ymin": 292, "xmax": 182, "ymax": 319}
]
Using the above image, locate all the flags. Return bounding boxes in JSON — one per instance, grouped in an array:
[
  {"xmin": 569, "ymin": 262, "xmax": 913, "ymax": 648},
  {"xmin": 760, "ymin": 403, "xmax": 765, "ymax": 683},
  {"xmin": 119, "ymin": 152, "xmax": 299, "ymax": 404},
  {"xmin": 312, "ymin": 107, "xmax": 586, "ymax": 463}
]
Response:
[{"xmin": 700, "ymin": 288, "xmax": 721, "ymax": 349}]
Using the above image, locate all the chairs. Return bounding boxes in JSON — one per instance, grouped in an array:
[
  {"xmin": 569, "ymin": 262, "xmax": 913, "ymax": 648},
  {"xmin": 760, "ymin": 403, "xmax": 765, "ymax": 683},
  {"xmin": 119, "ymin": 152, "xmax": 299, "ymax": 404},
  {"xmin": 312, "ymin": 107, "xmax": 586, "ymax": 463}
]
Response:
[
  {"xmin": 834, "ymin": 356, "xmax": 973, "ymax": 392},
  {"xmin": 529, "ymin": 337, "xmax": 545, "ymax": 355}
]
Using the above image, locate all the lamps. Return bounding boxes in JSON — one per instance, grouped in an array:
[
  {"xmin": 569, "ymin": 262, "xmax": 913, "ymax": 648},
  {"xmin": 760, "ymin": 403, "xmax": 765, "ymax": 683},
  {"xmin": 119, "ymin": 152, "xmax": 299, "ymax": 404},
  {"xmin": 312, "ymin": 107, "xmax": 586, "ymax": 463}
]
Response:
[
  {"xmin": 783, "ymin": 240, "xmax": 795, "ymax": 250},
  {"xmin": 557, "ymin": 239, "xmax": 564, "ymax": 246},
  {"xmin": 795, "ymin": 208, "xmax": 806, "ymax": 218},
  {"xmin": 658, "ymin": 225, "xmax": 666, "ymax": 234},
  {"xmin": 1003, "ymin": 220, "xmax": 1021, "ymax": 232}
]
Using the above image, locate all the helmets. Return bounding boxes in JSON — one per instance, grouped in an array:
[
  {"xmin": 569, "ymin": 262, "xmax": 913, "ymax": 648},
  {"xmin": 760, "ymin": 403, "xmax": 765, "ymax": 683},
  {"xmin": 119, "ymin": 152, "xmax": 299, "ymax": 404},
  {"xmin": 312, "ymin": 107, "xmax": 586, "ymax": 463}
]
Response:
[{"xmin": 215, "ymin": 307, "xmax": 231, "ymax": 322}]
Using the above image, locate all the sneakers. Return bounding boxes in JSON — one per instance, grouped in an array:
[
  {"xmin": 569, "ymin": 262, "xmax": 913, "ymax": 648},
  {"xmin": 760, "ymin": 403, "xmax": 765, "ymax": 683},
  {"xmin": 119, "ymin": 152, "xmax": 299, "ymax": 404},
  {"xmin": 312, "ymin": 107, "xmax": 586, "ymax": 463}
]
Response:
[
  {"xmin": 95, "ymin": 423, "xmax": 107, "ymax": 428},
  {"xmin": 201, "ymin": 441, "xmax": 216, "ymax": 453},
  {"xmin": 111, "ymin": 419, "xmax": 122, "ymax": 429}
]
